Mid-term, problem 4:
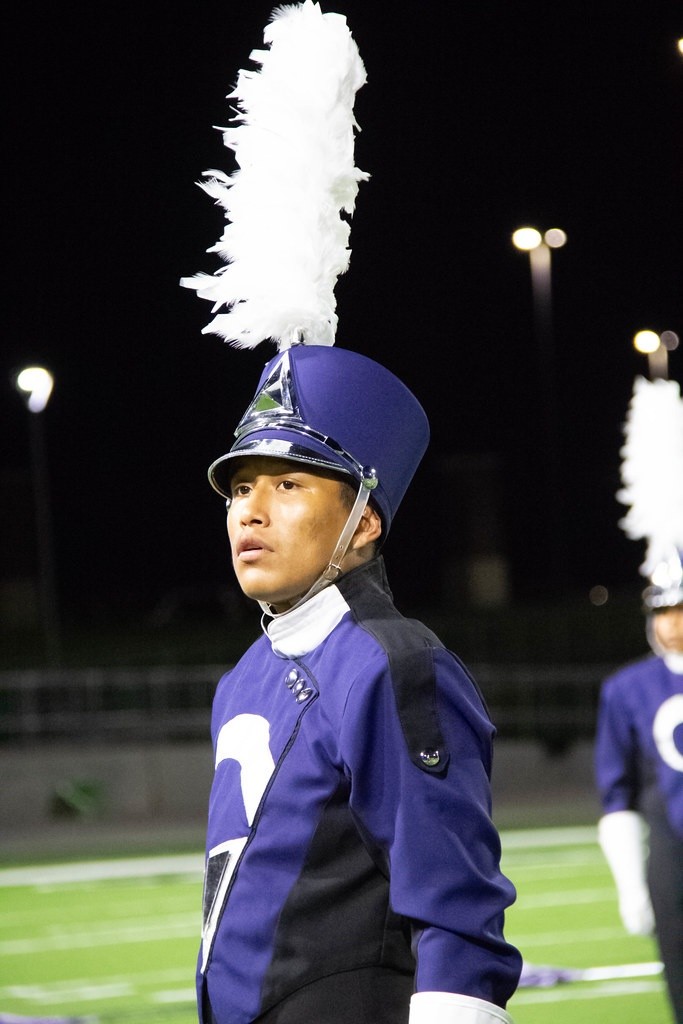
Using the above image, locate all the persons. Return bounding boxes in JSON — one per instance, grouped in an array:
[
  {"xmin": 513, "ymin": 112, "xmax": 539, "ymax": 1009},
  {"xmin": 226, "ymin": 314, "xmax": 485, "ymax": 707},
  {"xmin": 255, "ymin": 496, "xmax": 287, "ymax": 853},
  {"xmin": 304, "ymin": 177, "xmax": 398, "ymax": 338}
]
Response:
[
  {"xmin": 196, "ymin": 341, "xmax": 525, "ymax": 1024},
  {"xmin": 595, "ymin": 556, "xmax": 683, "ymax": 1024}
]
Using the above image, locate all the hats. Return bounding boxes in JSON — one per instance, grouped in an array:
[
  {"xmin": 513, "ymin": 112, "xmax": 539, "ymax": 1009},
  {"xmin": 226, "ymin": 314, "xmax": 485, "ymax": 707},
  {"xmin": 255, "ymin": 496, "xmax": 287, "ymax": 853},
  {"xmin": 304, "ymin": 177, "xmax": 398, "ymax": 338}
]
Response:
[
  {"xmin": 642, "ymin": 547, "xmax": 682, "ymax": 613},
  {"xmin": 207, "ymin": 345, "xmax": 431, "ymax": 556}
]
